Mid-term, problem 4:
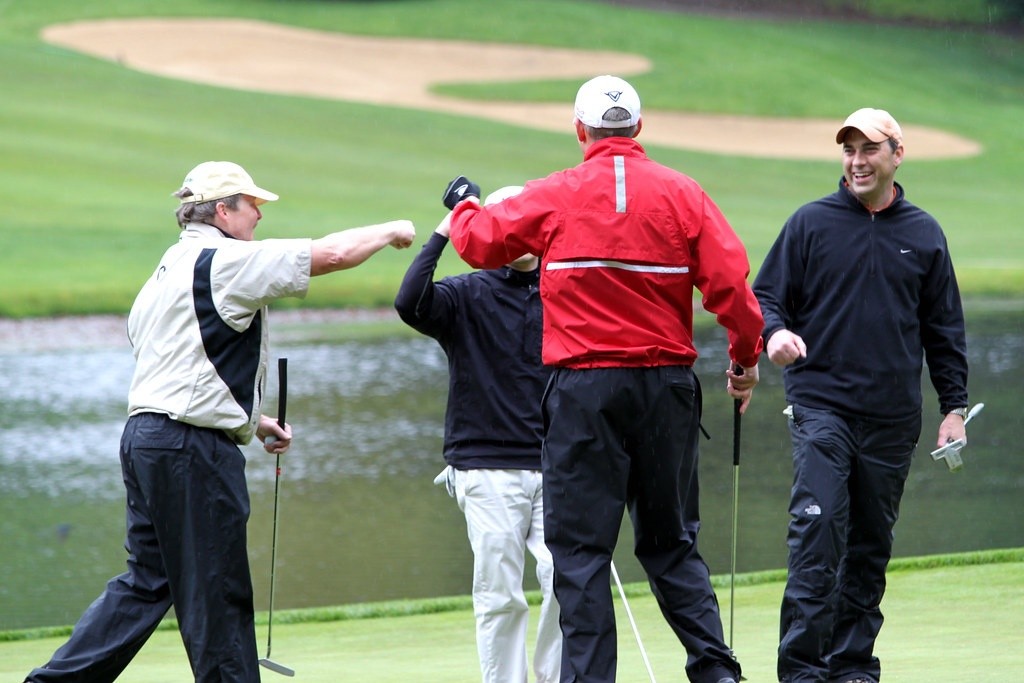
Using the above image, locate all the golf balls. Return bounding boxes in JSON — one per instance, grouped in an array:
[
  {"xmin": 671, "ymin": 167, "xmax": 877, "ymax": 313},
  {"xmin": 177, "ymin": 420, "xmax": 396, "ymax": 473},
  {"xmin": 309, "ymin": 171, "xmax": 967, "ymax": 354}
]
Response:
[{"xmin": 264, "ymin": 435, "xmax": 277, "ymax": 446}]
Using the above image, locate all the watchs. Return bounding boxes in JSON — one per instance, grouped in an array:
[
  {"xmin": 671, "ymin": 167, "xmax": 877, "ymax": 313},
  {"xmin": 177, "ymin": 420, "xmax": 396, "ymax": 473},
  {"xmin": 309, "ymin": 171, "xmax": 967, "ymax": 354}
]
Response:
[{"xmin": 949, "ymin": 407, "xmax": 966, "ymax": 418}]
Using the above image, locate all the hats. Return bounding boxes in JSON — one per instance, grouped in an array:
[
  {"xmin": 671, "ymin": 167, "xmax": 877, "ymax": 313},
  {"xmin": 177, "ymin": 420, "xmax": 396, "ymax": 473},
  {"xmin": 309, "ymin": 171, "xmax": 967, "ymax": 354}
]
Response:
[
  {"xmin": 482, "ymin": 186, "xmax": 525, "ymax": 206},
  {"xmin": 573, "ymin": 75, "xmax": 641, "ymax": 130},
  {"xmin": 180, "ymin": 160, "xmax": 279, "ymax": 207},
  {"xmin": 835, "ymin": 107, "xmax": 903, "ymax": 145}
]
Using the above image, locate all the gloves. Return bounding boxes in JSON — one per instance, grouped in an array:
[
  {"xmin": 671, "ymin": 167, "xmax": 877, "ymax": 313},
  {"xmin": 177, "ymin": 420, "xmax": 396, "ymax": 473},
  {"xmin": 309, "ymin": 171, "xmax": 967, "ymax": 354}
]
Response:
[{"xmin": 442, "ymin": 175, "xmax": 481, "ymax": 211}]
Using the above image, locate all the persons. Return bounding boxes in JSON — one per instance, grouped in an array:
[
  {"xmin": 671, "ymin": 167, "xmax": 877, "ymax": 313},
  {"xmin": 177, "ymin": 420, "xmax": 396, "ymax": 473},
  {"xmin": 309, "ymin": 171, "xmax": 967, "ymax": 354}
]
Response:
[
  {"xmin": 23, "ymin": 160, "xmax": 415, "ymax": 683},
  {"xmin": 452, "ymin": 72, "xmax": 764, "ymax": 683},
  {"xmin": 751, "ymin": 109, "xmax": 969, "ymax": 683},
  {"xmin": 395, "ymin": 185, "xmax": 567, "ymax": 682}
]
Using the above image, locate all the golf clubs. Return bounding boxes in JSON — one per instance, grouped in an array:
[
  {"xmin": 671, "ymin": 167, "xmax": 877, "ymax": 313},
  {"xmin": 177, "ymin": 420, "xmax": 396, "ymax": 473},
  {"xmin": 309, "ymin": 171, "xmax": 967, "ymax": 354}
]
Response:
[
  {"xmin": 258, "ymin": 355, "xmax": 297, "ymax": 677},
  {"xmin": 931, "ymin": 402, "xmax": 985, "ymax": 471},
  {"xmin": 730, "ymin": 366, "xmax": 750, "ymax": 683},
  {"xmin": 610, "ymin": 562, "xmax": 659, "ymax": 683}
]
